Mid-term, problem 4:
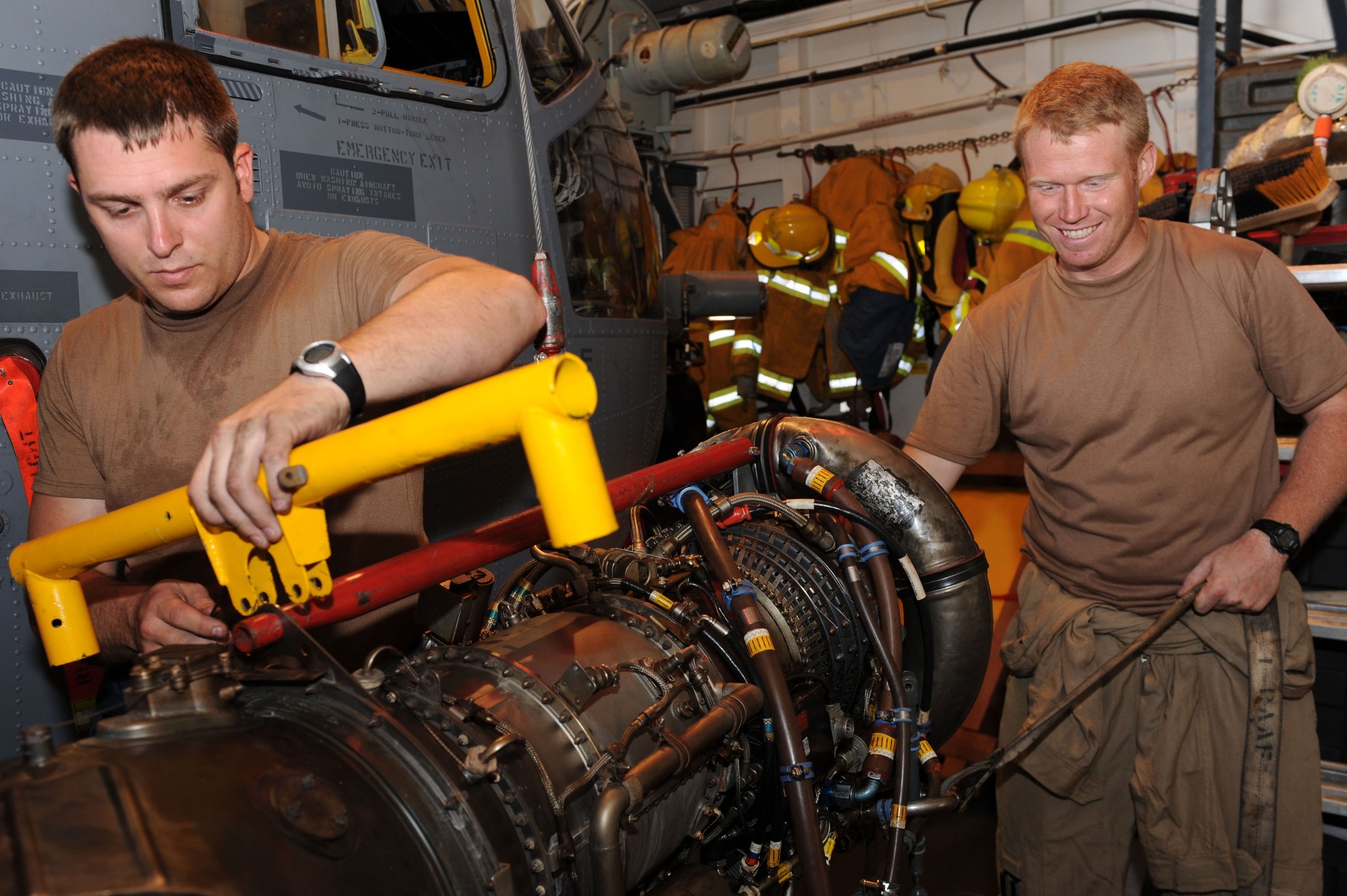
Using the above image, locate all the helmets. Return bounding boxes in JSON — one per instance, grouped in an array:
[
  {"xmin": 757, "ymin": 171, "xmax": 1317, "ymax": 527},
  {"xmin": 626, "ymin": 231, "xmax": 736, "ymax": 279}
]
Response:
[
  {"xmin": 957, "ymin": 165, "xmax": 1027, "ymax": 245},
  {"xmin": 748, "ymin": 203, "xmax": 830, "ymax": 268},
  {"xmin": 900, "ymin": 162, "xmax": 963, "ymax": 222}
]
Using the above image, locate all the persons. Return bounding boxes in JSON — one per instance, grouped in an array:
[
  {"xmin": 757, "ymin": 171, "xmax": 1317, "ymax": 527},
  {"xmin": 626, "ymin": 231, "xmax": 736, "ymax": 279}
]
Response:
[
  {"xmin": 910, "ymin": 61, "xmax": 1346, "ymax": 894},
  {"xmin": 21, "ymin": 37, "xmax": 547, "ymax": 678}
]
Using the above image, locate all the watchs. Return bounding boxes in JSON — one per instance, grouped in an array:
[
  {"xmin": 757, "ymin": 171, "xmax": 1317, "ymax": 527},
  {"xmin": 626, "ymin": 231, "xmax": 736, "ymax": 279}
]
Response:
[
  {"xmin": 285, "ymin": 338, "xmax": 367, "ymax": 428},
  {"xmin": 1250, "ymin": 517, "xmax": 1305, "ymax": 563}
]
